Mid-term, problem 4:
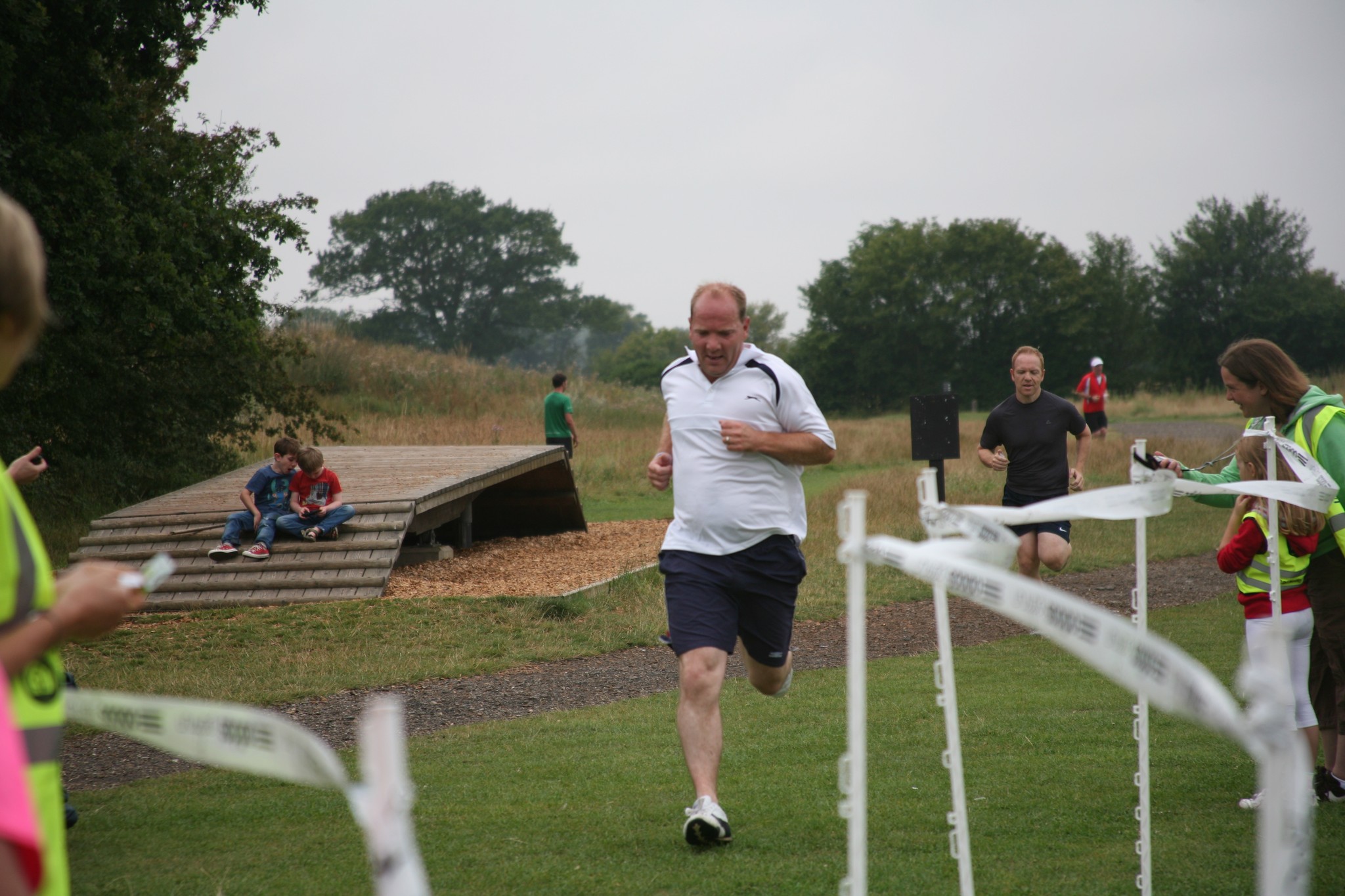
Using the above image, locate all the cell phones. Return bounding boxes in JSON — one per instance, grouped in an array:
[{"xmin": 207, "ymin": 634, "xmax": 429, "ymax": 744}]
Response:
[{"xmin": 301, "ymin": 510, "xmax": 322, "ymax": 516}]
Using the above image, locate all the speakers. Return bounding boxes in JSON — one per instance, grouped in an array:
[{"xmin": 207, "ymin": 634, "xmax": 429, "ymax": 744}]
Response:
[{"xmin": 909, "ymin": 393, "xmax": 961, "ymax": 462}]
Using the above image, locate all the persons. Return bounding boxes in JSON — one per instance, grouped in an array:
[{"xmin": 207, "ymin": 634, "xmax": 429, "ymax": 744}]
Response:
[
  {"xmin": 1076, "ymin": 356, "xmax": 1111, "ymax": 438},
  {"xmin": 207, "ymin": 436, "xmax": 303, "ymax": 559},
  {"xmin": 977, "ymin": 346, "xmax": 1093, "ymax": 583},
  {"xmin": 0, "ymin": 195, "xmax": 147, "ymax": 896},
  {"xmin": 273, "ymin": 445, "xmax": 356, "ymax": 543},
  {"xmin": 5, "ymin": 446, "xmax": 48, "ymax": 487},
  {"xmin": 645, "ymin": 280, "xmax": 840, "ymax": 845},
  {"xmin": 1215, "ymin": 429, "xmax": 1324, "ymax": 811},
  {"xmin": 1152, "ymin": 336, "xmax": 1345, "ymax": 805},
  {"xmin": 543, "ymin": 373, "xmax": 579, "ymax": 459}
]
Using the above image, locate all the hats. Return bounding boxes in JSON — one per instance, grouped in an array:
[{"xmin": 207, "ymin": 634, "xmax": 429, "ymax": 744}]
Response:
[{"xmin": 1090, "ymin": 356, "xmax": 1104, "ymax": 367}]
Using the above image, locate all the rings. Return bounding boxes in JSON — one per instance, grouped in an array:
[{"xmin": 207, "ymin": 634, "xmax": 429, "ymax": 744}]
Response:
[{"xmin": 725, "ymin": 435, "xmax": 730, "ymax": 443}]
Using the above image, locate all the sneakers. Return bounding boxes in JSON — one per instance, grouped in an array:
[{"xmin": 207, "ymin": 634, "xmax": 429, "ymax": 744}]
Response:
[
  {"xmin": 1236, "ymin": 787, "xmax": 1320, "ymax": 811},
  {"xmin": 681, "ymin": 794, "xmax": 734, "ymax": 851},
  {"xmin": 771, "ymin": 667, "xmax": 793, "ymax": 699},
  {"xmin": 1312, "ymin": 765, "xmax": 1345, "ymax": 806},
  {"xmin": 242, "ymin": 544, "xmax": 269, "ymax": 559},
  {"xmin": 208, "ymin": 544, "xmax": 239, "ymax": 561}
]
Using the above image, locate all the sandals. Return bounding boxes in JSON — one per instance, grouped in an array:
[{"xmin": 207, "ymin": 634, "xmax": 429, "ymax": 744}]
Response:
[
  {"xmin": 327, "ymin": 527, "xmax": 339, "ymax": 542},
  {"xmin": 300, "ymin": 527, "xmax": 319, "ymax": 542}
]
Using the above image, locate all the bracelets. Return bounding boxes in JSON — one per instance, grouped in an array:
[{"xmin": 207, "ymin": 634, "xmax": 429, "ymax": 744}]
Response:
[{"xmin": 655, "ymin": 452, "xmax": 672, "ymax": 461}]
